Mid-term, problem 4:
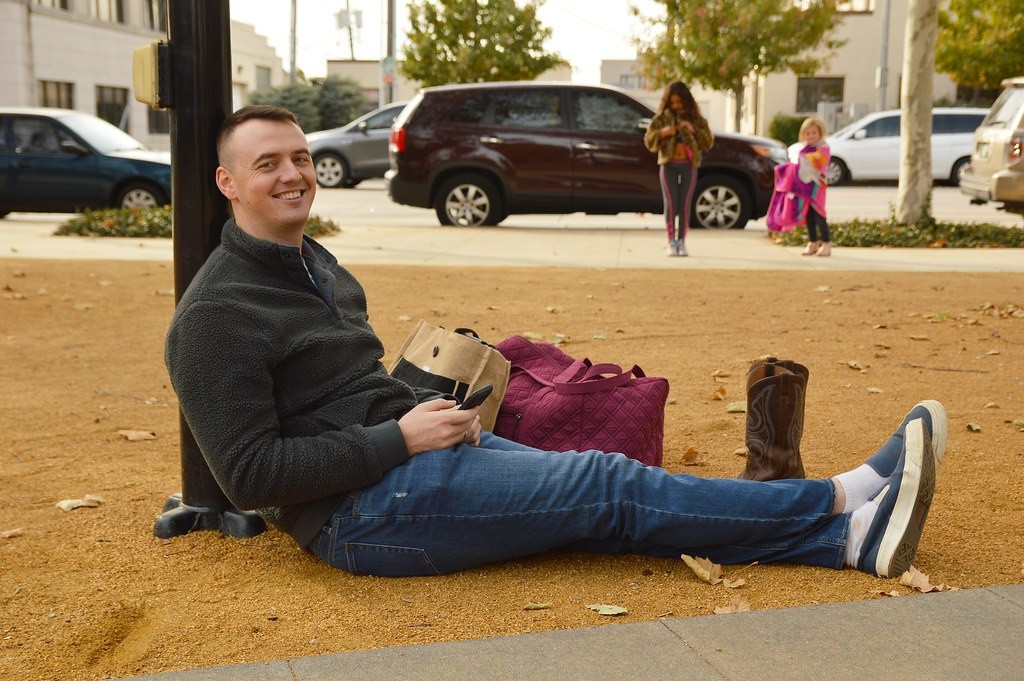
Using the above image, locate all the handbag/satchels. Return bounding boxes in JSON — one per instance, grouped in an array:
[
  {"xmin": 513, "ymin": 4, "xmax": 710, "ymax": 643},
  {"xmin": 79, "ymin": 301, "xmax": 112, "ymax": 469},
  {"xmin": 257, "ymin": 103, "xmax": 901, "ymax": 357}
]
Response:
[
  {"xmin": 492, "ymin": 335, "xmax": 670, "ymax": 467},
  {"xmin": 386, "ymin": 319, "xmax": 511, "ymax": 434}
]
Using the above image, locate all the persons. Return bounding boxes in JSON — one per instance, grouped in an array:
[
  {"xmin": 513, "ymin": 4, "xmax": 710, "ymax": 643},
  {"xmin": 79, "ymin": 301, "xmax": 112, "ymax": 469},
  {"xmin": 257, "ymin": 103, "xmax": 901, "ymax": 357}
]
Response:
[
  {"xmin": 170, "ymin": 106, "xmax": 949, "ymax": 578},
  {"xmin": 644, "ymin": 82, "xmax": 714, "ymax": 257},
  {"xmin": 797, "ymin": 117, "xmax": 830, "ymax": 257}
]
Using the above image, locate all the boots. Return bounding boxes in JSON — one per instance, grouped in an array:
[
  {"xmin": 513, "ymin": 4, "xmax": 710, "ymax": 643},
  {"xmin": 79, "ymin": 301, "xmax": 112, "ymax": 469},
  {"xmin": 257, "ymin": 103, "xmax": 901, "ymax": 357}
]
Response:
[{"xmin": 736, "ymin": 357, "xmax": 809, "ymax": 483}]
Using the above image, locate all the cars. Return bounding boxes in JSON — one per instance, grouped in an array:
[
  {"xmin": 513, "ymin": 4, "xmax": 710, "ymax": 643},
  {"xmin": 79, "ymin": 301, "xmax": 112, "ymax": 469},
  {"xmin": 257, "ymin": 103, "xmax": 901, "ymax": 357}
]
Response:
[
  {"xmin": 303, "ymin": 101, "xmax": 407, "ymax": 187},
  {"xmin": 0, "ymin": 107, "xmax": 173, "ymax": 219}
]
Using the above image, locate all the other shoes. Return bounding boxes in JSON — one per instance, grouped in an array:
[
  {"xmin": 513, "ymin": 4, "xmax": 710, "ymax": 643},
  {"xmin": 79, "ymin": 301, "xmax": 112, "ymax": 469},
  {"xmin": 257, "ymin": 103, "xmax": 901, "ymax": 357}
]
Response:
[
  {"xmin": 802, "ymin": 241, "xmax": 819, "ymax": 255},
  {"xmin": 815, "ymin": 241, "xmax": 832, "ymax": 256}
]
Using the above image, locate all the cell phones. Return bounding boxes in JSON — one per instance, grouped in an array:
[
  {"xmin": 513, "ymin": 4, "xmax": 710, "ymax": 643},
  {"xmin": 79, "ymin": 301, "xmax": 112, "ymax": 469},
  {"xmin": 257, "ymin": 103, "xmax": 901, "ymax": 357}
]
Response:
[{"xmin": 458, "ymin": 384, "xmax": 493, "ymax": 410}]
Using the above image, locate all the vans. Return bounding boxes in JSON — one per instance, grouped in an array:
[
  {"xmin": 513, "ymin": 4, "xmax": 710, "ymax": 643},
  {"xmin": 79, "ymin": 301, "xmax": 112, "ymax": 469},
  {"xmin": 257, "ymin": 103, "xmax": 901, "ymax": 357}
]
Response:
[{"xmin": 789, "ymin": 106, "xmax": 989, "ymax": 188}]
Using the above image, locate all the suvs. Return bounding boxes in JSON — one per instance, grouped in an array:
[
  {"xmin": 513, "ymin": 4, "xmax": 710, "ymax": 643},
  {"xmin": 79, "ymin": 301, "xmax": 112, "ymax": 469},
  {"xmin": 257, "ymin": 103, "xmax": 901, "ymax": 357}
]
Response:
[
  {"xmin": 386, "ymin": 81, "xmax": 789, "ymax": 230},
  {"xmin": 957, "ymin": 76, "xmax": 1024, "ymax": 214}
]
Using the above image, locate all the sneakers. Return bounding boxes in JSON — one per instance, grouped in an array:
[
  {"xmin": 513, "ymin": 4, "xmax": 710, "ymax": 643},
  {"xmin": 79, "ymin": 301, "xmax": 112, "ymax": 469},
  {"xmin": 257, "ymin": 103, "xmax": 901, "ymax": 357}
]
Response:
[
  {"xmin": 668, "ymin": 238, "xmax": 688, "ymax": 256},
  {"xmin": 855, "ymin": 399, "xmax": 948, "ymax": 579}
]
